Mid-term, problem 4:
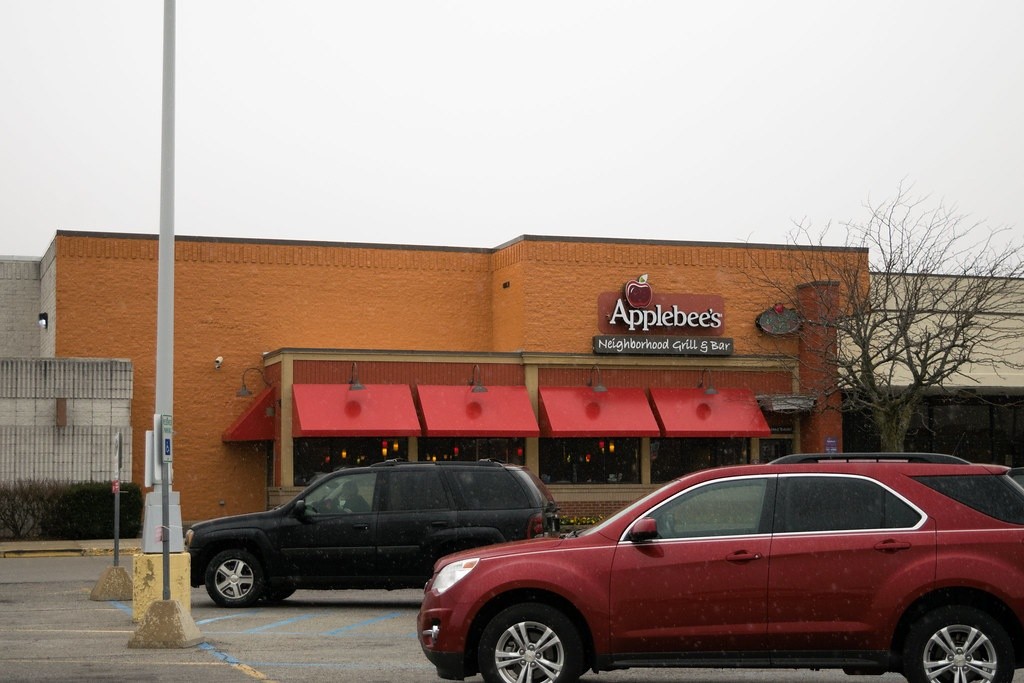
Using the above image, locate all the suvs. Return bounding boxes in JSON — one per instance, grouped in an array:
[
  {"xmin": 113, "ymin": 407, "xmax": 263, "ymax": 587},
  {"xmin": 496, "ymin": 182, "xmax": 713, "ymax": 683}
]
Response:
[
  {"xmin": 414, "ymin": 452, "xmax": 1024, "ymax": 683},
  {"xmin": 183, "ymin": 456, "xmax": 563, "ymax": 609}
]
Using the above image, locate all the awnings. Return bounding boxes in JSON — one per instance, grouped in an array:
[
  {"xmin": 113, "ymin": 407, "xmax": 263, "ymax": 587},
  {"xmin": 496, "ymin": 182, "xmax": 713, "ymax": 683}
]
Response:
[
  {"xmin": 417, "ymin": 384, "xmax": 539, "ymax": 438},
  {"xmin": 221, "ymin": 387, "xmax": 276, "ymax": 442},
  {"xmin": 648, "ymin": 386, "xmax": 771, "ymax": 438},
  {"xmin": 538, "ymin": 386, "xmax": 661, "ymax": 437},
  {"xmin": 292, "ymin": 383, "xmax": 421, "ymax": 437}
]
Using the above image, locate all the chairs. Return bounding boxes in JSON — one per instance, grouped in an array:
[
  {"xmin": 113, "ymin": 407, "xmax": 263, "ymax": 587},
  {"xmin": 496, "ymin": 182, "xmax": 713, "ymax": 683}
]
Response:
[{"xmin": 342, "ymin": 481, "xmax": 370, "ymax": 513}]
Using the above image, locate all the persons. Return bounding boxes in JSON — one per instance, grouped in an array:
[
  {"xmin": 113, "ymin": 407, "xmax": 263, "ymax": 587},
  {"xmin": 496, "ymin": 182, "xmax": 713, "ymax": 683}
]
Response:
[{"xmin": 342, "ymin": 481, "xmax": 370, "ymax": 513}]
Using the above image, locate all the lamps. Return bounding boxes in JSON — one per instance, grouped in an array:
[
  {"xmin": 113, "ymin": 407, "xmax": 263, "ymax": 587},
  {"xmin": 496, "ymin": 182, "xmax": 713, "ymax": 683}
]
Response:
[
  {"xmin": 469, "ymin": 363, "xmax": 488, "ymax": 393},
  {"xmin": 697, "ymin": 367, "xmax": 719, "ymax": 394},
  {"xmin": 235, "ymin": 368, "xmax": 272, "ymax": 396},
  {"xmin": 349, "ymin": 362, "xmax": 367, "ymax": 390},
  {"xmin": 590, "ymin": 365, "xmax": 608, "ymax": 392}
]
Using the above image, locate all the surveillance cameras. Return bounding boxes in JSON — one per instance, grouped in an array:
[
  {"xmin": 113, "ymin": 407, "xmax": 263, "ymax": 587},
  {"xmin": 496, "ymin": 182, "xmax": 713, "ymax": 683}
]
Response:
[{"xmin": 214, "ymin": 356, "xmax": 223, "ymax": 364}]
